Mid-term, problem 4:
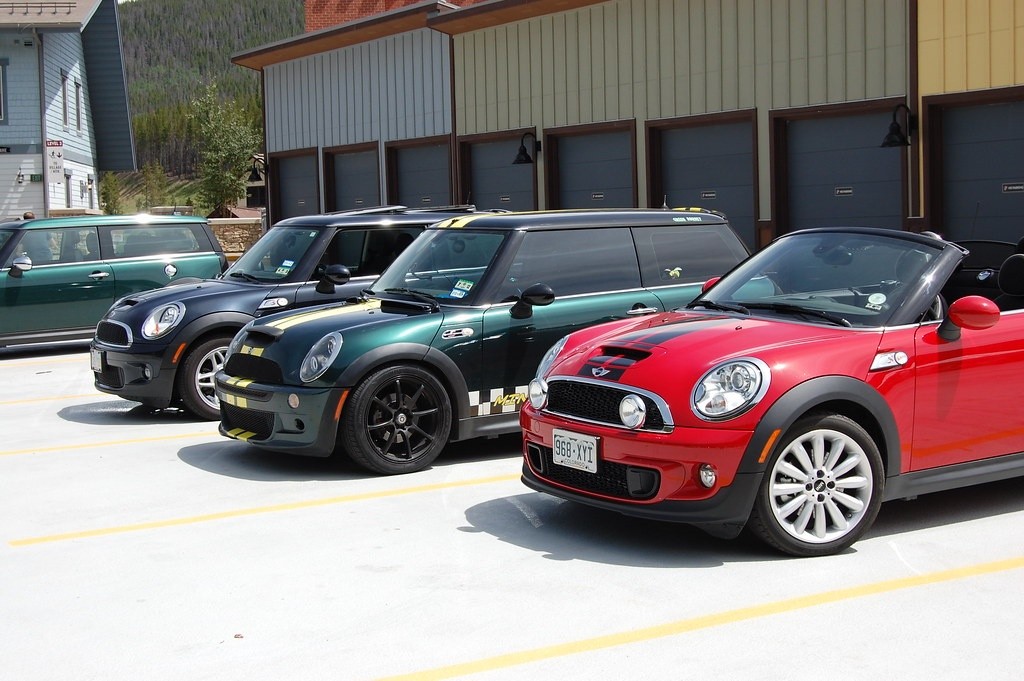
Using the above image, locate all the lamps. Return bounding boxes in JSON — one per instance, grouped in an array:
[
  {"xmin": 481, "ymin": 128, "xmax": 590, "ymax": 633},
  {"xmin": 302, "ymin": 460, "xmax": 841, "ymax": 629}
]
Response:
[
  {"xmin": 512, "ymin": 132, "xmax": 541, "ymax": 164},
  {"xmin": 246, "ymin": 158, "xmax": 270, "ymax": 182},
  {"xmin": 880, "ymin": 103, "xmax": 918, "ymax": 147}
]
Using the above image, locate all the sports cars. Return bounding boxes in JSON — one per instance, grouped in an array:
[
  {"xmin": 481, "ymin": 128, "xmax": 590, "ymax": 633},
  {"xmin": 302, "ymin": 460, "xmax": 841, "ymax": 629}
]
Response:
[{"xmin": 520, "ymin": 224, "xmax": 1024, "ymax": 556}]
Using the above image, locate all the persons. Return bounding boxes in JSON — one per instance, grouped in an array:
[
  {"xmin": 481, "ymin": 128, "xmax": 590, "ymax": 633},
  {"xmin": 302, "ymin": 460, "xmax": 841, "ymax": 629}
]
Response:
[{"xmin": 21, "ymin": 211, "xmax": 59, "ymax": 265}]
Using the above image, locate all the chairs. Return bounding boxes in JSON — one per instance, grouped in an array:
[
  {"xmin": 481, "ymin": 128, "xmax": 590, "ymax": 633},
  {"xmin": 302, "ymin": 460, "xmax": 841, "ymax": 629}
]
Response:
[
  {"xmin": 61, "ymin": 232, "xmax": 97, "ymax": 261},
  {"xmin": 355, "ymin": 232, "xmax": 413, "ymax": 274},
  {"xmin": 873, "ymin": 230, "xmax": 945, "ymax": 323},
  {"xmin": 991, "ymin": 254, "xmax": 1024, "ymax": 311}
]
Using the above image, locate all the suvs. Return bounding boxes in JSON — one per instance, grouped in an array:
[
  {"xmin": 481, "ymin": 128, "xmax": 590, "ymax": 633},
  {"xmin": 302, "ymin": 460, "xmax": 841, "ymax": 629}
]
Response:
[
  {"xmin": 90, "ymin": 202, "xmax": 515, "ymax": 421},
  {"xmin": 0, "ymin": 212, "xmax": 228, "ymax": 357},
  {"xmin": 215, "ymin": 204, "xmax": 776, "ymax": 474}
]
